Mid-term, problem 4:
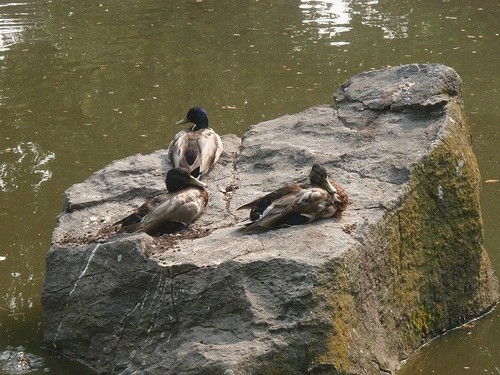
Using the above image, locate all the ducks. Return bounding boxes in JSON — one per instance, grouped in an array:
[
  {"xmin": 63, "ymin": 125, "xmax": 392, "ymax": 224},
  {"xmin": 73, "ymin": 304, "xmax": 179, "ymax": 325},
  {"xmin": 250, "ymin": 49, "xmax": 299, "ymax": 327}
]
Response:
[
  {"xmin": 112, "ymin": 167, "xmax": 208, "ymax": 238},
  {"xmin": 233, "ymin": 162, "xmax": 349, "ymax": 236},
  {"xmin": 166, "ymin": 106, "xmax": 223, "ymax": 180}
]
